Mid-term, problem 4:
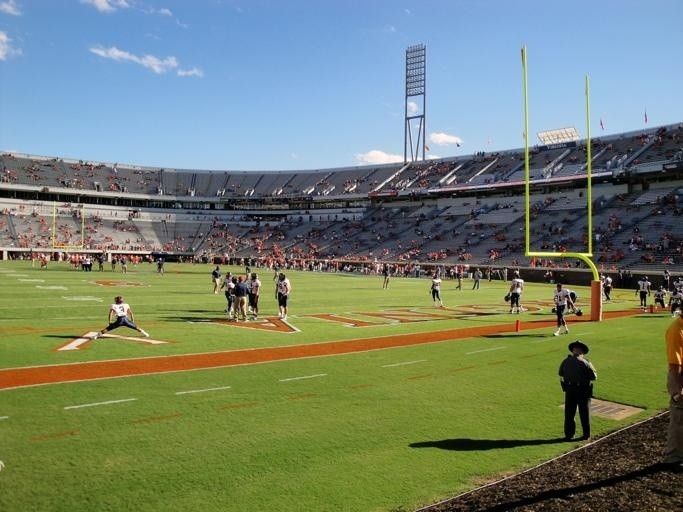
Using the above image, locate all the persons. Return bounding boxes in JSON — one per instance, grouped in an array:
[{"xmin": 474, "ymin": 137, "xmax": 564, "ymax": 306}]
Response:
[
  {"xmin": 1, "ymin": 126, "xmax": 683, "ymax": 338},
  {"xmin": 665, "ymin": 307, "xmax": 683, "ymax": 473},
  {"xmin": 559, "ymin": 341, "xmax": 597, "ymax": 441}
]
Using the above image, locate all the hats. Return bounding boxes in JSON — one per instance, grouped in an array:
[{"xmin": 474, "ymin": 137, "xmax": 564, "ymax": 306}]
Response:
[{"xmin": 569, "ymin": 341, "xmax": 588, "ymax": 354}]
